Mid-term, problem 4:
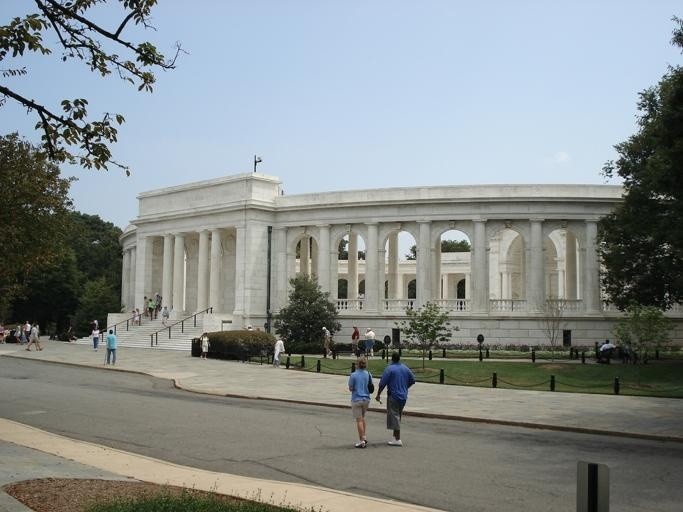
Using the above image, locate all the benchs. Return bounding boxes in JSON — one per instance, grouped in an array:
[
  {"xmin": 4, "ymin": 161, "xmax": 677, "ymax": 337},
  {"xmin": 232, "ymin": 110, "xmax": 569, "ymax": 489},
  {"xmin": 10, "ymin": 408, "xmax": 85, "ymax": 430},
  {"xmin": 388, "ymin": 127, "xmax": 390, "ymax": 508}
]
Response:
[{"xmin": 597, "ymin": 346, "xmax": 626, "ymax": 364}]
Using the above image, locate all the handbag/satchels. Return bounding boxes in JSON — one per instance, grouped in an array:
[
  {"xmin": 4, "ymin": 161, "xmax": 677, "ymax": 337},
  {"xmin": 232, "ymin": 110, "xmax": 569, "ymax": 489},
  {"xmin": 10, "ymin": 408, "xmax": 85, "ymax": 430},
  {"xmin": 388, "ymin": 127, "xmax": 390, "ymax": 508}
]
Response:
[{"xmin": 368, "ymin": 384, "xmax": 374, "ymax": 393}]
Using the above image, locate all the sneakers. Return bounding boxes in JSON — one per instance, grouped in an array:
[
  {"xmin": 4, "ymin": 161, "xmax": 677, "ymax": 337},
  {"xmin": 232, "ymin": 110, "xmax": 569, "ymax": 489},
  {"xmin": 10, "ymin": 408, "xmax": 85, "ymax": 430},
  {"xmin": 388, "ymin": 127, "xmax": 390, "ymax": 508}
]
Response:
[
  {"xmin": 356, "ymin": 440, "xmax": 367, "ymax": 448},
  {"xmin": 388, "ymin": 438, "xmax": 402, "ymax": 445}
]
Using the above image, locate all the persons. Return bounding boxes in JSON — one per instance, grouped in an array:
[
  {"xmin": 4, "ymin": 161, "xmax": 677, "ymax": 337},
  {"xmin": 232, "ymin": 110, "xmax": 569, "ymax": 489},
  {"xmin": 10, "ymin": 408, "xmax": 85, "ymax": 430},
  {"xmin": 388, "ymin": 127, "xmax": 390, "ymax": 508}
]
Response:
[
  {"xmin": 105, "ymin": 329, "xmax": 117, "ymax": 365},
  {"xmin": 272, "ymin": 337, "xmax": 285, "ymax": 368},
  {"xmin": 348, "ymin": 356, "xmax": 373, "ymax": 448},
  {"xmin": 132, "ymin": 292, "xmax": 169, "ymax": 329},
  {"xmin": 0, "ymin": 320, "xmax": 44, "ymax": 351},
  {"xmin": 350, "ymin": 325, "xmax": 360, "ymax": 356},
  {"xmin": 321, "ymin": 326, "xmax": 332, "ymax": 359},
  {"xmin": 90, "ymin": 326, "xmax": 99, "ymax": 351},
  {"xmin": 599, "ymin": 339, "xmax": 616, "ymax": 363},
  {"xmin": 199, "ymin": 332, "xmax": 210, "ymax": 361},
  {"xmin": 374, "ymin": 352, "xmax": 416, "ymax": 446},
  {"xmin": 623, "ymin": 339, "xmax": 633, "ymax": 365},
  {"xmin": 364, "ymin": 327, "xmax": 377, "ymax": 358}
]
